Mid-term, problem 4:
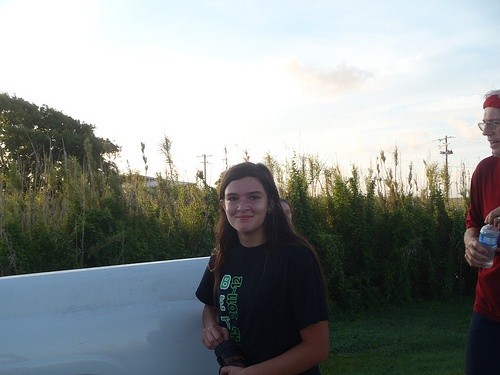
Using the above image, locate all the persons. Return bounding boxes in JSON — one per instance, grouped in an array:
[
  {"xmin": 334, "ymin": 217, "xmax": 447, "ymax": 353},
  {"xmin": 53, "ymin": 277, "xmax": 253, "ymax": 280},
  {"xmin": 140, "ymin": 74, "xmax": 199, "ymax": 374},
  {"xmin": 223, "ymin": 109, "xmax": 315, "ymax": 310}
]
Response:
[
  {"xmin": 277, "ymin": 197, "xmax": 292, "ymax": 223},
  {"xmin": 462, "ymin": 87, "xmax": 500, "ymax": 375},
  {"xmin": 198, "ymin": 162, "xmax": 330, "ymax": 375}
]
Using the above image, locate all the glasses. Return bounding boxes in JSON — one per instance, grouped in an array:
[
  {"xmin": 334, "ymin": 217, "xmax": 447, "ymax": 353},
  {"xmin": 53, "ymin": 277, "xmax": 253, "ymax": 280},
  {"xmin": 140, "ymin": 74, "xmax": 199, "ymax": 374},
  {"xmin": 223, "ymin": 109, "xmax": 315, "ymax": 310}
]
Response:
[{"xmin": 478, "ymin": 121, "xmax": 500, "ymax": 131}]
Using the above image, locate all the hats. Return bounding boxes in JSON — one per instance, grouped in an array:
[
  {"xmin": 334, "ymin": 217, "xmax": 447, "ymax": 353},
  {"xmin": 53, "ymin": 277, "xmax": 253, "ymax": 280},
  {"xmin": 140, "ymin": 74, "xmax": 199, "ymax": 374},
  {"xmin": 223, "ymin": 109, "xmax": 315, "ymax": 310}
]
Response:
[{"xmin": 483, "ymin": 94, "xmax": 500, "ymax": 109}]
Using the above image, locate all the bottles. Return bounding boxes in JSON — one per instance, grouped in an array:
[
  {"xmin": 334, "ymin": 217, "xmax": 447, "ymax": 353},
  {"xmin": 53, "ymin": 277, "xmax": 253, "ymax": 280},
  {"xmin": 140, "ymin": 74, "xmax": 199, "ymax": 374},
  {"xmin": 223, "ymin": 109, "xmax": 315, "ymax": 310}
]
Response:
[
  {"xmin": 215, "ymin": 339, "xmax": 245, "ymax": 368},
  {"xmin": 477, "ymin": 222, "xmax": 500, "ymax": 268}
]
